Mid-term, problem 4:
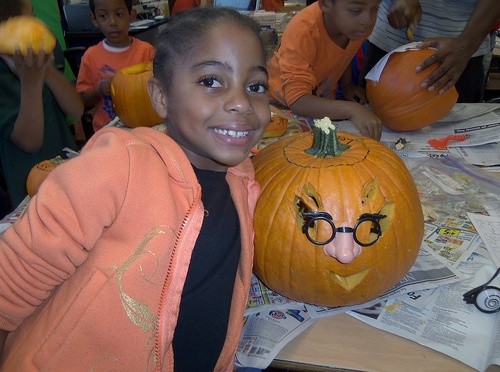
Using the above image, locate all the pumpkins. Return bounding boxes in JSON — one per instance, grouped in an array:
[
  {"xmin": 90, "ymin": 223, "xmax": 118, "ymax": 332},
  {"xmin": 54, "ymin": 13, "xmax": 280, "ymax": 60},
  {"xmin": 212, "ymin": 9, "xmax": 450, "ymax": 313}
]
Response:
[{"xmin": 365, "ymin": 47, "xmax": 458, "ymax": 131}]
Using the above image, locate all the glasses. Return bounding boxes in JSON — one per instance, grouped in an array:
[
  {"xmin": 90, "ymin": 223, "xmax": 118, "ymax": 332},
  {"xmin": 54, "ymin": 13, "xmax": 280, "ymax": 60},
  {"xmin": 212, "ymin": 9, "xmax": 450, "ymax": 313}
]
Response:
[
  {"xmin": 304, "ymin": 217, "xmax": 382, "ymax": 247},
  {"xmin": 463, "ymin": 266, "xmax": 499, "ymax": 316}
]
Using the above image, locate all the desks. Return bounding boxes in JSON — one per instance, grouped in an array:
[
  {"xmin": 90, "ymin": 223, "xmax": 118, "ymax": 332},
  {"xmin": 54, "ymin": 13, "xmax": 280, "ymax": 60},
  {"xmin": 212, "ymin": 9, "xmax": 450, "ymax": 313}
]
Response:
[{"xmin": 268, "ymin": 313, "xmax": 500, "ymax": 372}]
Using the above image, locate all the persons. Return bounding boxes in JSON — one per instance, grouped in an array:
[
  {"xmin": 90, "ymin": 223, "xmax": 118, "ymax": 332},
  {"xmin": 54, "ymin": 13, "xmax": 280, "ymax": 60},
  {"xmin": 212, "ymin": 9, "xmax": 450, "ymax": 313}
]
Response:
[
  {"xmin": 0, "ymin": 0, "xmax": 84, "ymax": 221},
  {"xmin": 76, "ymin": 0, "xmax": 157, "ymax": 134},
  {"xmin": 365, "ymin": 0, "xmax": 500, "ymax": 103},
  {"xmin": 0, "ymin": 7, "xmax": 271, "ymax": 372},
  {"xmin": 267, "ymin": 0, "xmax": 383, "ymax": 142}
]
